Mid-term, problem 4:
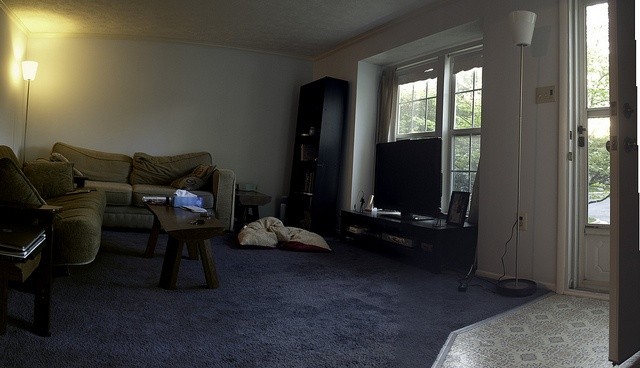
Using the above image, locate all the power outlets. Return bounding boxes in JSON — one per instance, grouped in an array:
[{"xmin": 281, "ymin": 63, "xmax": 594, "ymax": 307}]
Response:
[{"xmin": 519, "ymin": 213, "xmax": 528, "ymax": 232}]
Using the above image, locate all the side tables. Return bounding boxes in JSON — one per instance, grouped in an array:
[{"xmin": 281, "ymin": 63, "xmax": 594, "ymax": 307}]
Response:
[{"xmin": 235, "ymin": 189, "xmax": 272, "ymax": 236}]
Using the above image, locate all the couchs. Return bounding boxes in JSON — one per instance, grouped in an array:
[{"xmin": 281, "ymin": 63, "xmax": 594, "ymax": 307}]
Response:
[
  {"xmin": 32, "ymin": 143, "xmax": 235, "ymax": 281},
  {"xmin": 0, "ymin": 146, "xmax": 108, "ymax": 277}
]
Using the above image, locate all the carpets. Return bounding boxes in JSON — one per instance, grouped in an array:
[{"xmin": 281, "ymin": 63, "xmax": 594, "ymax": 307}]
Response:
[{"xmin": 0, "ymin": 228, "xmax": 555, "ymax": 368}]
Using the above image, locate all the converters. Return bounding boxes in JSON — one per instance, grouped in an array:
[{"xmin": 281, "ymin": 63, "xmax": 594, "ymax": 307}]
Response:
[{"xmin": 458, "ymin": 281, "xmax": 468, "ymax": 291}]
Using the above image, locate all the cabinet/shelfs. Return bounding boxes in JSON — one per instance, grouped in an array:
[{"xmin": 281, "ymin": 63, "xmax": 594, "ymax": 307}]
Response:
[
  {"xmin": 0, "ymin": 203, "xmax": 63, "ymax": 338},
  {"xmin": 289, "ymin": 76, "xmax": 349, "ymax": 236},
  {"xmin": 339, "ymin": 209, "xmax": 478, "ymax": 275}
]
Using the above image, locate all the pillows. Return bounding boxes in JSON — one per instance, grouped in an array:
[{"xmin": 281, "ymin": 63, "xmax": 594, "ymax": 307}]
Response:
[
  {"xmin": 268, "ymin": 225, "xmax": 332, "ymax": 252},
  {"xmin": 25, "ymin": 163, "xmax": 73, "ymax": 192},
  {"xmin": 51, "ymin": 142, "xmax": 130, "ymax": 183},
  {"xmin": 170, "ymin": 165, "xmax": 215, "ymax": 191},
  {"xmin": 0, "ymin": 158, "xmax": 48, "ymax": 206},
  {"xmin": 49, "ymin": 153, "xmax": 83, "ymax": 176},
  {"xmin": 238, "ymin": 216, "xmax": 288, "ymax": 248},
  {"xmin": 130, "ymin": 151, "xmax": 212, "ymax": 185}
]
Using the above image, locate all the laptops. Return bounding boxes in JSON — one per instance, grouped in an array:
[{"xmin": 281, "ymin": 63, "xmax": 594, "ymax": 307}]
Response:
[{"xmin": 0, "ymin": 223, "xmax": 46, "ymax": 255}]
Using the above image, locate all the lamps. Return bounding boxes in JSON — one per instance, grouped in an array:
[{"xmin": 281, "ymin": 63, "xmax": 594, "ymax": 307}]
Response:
[
  {"xmin": 21, "ymin": 60, "xmax": 38, "ymax": 167},
  {"xmin": 498, "ymin": 10, "xmax": 537, "ymax": 297}
]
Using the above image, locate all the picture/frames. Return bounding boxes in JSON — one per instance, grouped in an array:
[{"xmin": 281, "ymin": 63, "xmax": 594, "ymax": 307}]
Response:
[{"xmin": 447, "ymin": 191, "xmax": 470, "ymax": 227}]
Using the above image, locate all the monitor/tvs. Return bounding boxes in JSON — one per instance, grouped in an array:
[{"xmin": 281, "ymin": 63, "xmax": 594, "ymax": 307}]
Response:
[{"xmin": 374, "ymin": 138, "xmax": 442, "ymax": 221}]
